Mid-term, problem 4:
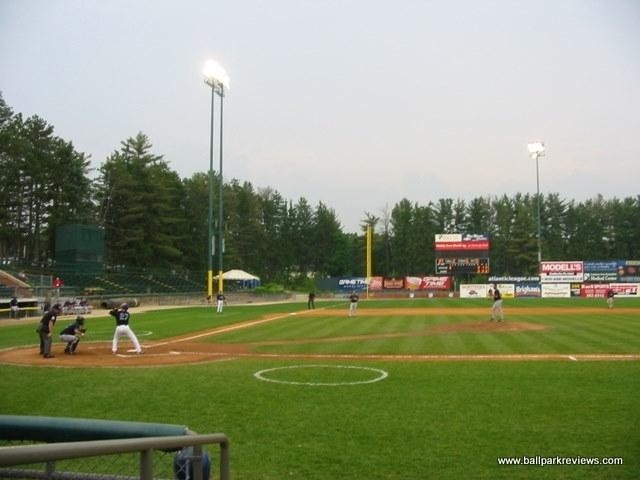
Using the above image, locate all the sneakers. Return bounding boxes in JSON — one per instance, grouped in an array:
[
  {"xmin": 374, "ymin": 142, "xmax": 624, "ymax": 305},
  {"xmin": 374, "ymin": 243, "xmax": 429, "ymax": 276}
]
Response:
[
  {"xmin": 111, "ymin": 349, "xmax": 145, "ymax": 355},
  {"xmin": 39, "ymin": 349, "xmax": 76, "ymax": 359}
]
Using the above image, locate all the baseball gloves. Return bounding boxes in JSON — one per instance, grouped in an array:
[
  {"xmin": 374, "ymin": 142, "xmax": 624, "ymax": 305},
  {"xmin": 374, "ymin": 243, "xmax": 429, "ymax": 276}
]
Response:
[{"xmin": 80, "ymin": 328, "xmax": 85, "ymax": 333}]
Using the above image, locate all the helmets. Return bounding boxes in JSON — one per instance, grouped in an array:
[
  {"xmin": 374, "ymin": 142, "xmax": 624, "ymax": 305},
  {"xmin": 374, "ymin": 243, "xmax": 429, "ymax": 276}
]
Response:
[{"xmin": 120, "ymin": 302, "xmax": 129, "ymax": 310}]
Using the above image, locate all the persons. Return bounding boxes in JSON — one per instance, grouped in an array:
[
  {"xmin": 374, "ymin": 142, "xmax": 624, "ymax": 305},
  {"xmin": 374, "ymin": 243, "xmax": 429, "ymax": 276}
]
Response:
[
  {"xmin": 9, "ymin": 298, "xmax": 19, "ymax": 313},
  {"xmin": 60, "ymin": 316, "xmax": 85, "ymax": 354},
  {"xmin": 491, "ymin": 282, "xmax": 504, "ymax": 322},
  {"xmin": 216, "ymin": 291, "xmax": 225, "ymax": 314},
  {"xmin": 349, "ymin": 290, "xmax": 359, "ymax": 317},
  {"xmin": 52, "ymin": 276, "xmax": 64, "ymax": 297},
  {"xmin": 36, "ymin": 304, "xmax": 61, "ymax": 358},
  {"xmin": 308, "ymin": 290, "xmax": 315, "ymax": 309},
  {"xmin": 17, "ymin": 270, "xmax": 28, "ymax": 282},
  {"xmin": 44, "ymin": 298, "xmax": 88, "ymax": 314},
  {"xmin": 607, "ymin": 289, "xmax": 614, "ymax": 308},
  {"xmin": 109, "ymin": 303, "xmax": 142, "ymax": 353}
]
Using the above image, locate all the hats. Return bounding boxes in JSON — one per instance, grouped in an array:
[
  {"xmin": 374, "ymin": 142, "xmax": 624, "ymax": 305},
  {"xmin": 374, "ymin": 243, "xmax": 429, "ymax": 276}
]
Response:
[{"xmin": 52, "ymin": 304, "xmax": 62, "ymax": 311}]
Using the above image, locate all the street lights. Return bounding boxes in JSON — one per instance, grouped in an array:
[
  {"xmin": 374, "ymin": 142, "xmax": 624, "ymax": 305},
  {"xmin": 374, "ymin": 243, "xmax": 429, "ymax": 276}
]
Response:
[
  {"xmin": 204, "ymin": 61, "xmax": 228, "ymax": 301},
  {"xmin": 528, "ymin": 142, "xmax": 548, "ymax": 263}
]
